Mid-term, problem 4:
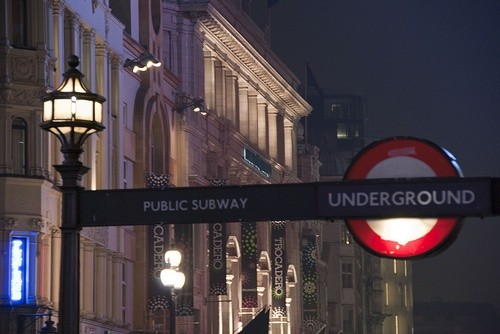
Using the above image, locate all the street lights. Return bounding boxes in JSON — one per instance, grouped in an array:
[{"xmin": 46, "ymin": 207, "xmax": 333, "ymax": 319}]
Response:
[
  {"xmin": 156, "ymin": 240, "xmax": 186, "ymax": 334},
  {"xmin": 39, "ymin": 52, "xmax": 106, "ymax": 334}
]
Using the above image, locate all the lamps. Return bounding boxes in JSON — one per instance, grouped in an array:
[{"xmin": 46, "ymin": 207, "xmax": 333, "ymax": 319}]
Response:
[
  {"xmin": 172, "ymin": 95, "xmax": 207, "ymax": 116},
  {"xmin": 123, "ymin": 51, "xmax": 161, "ymax": 73}
]
